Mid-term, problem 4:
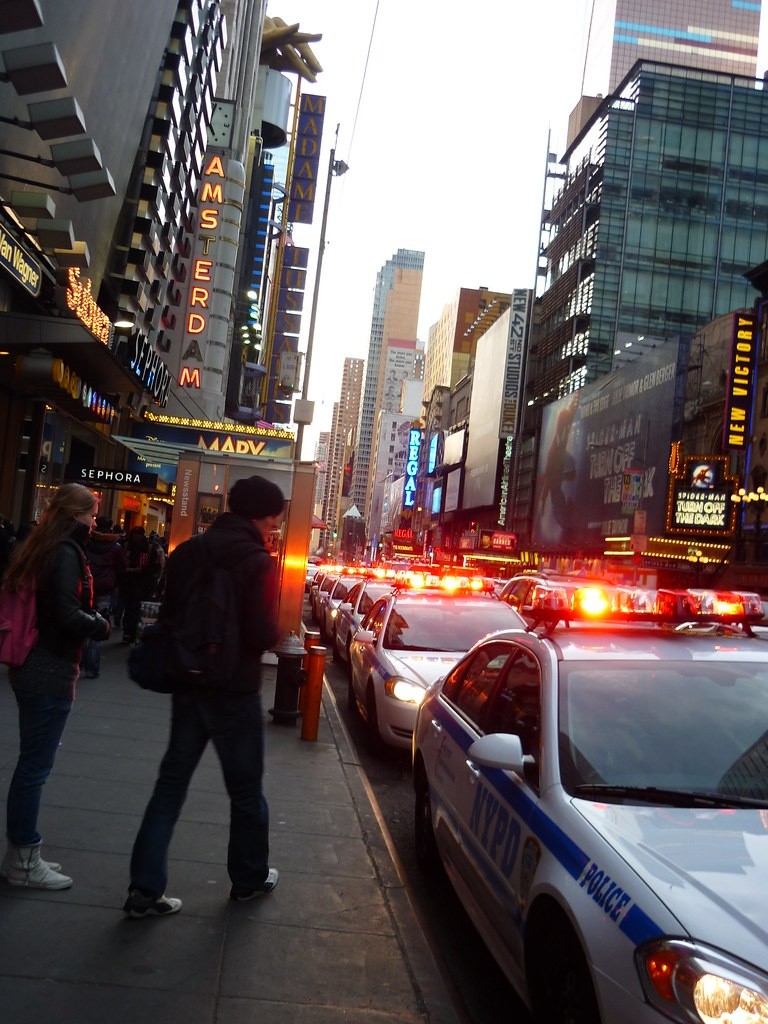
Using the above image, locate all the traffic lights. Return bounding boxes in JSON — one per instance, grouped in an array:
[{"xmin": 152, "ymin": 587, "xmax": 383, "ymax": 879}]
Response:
[{"xmin": 344, "ymin": 464, "xmax": 352, "ymax": 484}]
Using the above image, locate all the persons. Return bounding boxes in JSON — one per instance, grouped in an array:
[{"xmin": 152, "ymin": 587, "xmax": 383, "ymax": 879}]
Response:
[
  {"xmin": 0, "ymin": 482, "xmax": 113, "ymax": 889},
  {"xmin": 124, "ymin": 475, "xmax": 286, "ymax": 918},
  {"xmin": 1, "ymin": 513, "xmax": 168, "ymax": 680}
]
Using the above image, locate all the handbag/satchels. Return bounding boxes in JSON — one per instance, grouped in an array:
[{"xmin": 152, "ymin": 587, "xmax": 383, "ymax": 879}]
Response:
[{"xmin": 140, "ymin": 553, "xmax": 149, "ymax": 568}]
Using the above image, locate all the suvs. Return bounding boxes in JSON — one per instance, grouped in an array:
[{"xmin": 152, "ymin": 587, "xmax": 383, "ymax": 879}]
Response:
[{"xmin": 498, "ymin": 570, "xmax": 615, "ymax": 625}]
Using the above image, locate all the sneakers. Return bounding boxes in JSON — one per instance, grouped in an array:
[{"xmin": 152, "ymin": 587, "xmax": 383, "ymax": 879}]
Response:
[
  {"xmin": 123, "ymin": 892, "xmax": 185, "ymax": 918},
  {"xmin": 122, "ymin": 632, "xmax": 133, "ymax": 644},
  {"xmin": 230, "ymin": 867, "xmax": 279, "ymax": 901}
]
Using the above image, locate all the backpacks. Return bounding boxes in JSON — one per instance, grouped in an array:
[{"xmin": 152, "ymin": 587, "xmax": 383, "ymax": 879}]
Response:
[
  {"xmin": 129, "ymin": 538, "xmax": 267, "ymax": 693},
  {"xmin": 150, "ymin": 540, "xmax": 165, "ymax": 579},
  {"xmin": 97, "ymin": 544, "xmax": 120, "ymax": 592},
  {"xmin": 0, "ymin": 537, "xmax": 95, "ymax": 669}
]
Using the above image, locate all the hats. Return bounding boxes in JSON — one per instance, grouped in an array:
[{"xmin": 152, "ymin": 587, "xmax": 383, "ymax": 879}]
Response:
[{"xmin": 228, "ymin": 475, "xmax": 284, "ymax": 516}]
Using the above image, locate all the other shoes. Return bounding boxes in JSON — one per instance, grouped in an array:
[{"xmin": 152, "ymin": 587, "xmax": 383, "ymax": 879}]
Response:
[{"xmin": 85, "ymin": 671, "xmax": 99, "ymax": 678}]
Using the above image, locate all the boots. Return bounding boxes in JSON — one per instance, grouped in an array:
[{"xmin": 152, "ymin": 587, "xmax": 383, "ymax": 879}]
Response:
[{"xmin": 0, "ymin": 835, "xmax": 74, "ymax": 891}]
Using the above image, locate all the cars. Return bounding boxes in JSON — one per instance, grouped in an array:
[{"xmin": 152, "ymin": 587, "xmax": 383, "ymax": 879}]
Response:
[
  {"xmin": 411, "ymin": 586, "xmax": 768, "ymax": 1024},
  {"xmin": 318, "ymin": 569, "xmax": 373, "ymax": 644},
  {"xmin": 334, "ymin": 569, "xmax": 432, "ymax": 665},
  {"xmin": 348, "ymin": 574, "xmax": 534, "ymax": 750},
  {"xmin": 309, "ymin": 565, "xmax": 335, "ymax": 608}
]
